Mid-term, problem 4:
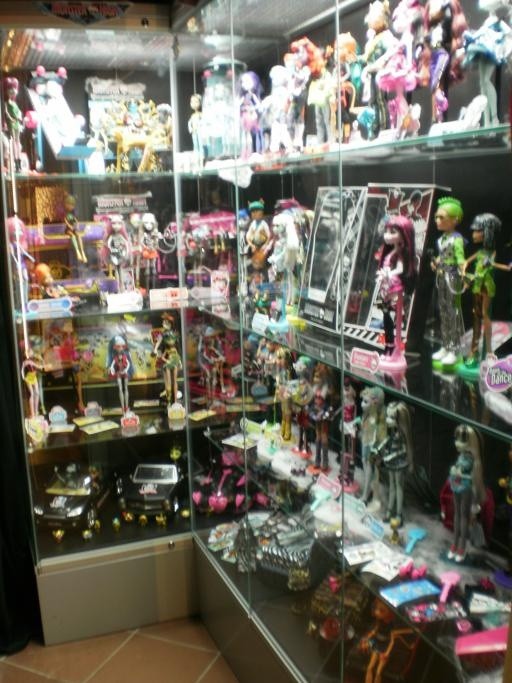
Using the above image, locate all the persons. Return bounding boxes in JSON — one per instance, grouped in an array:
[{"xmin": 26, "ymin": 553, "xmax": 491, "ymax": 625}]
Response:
[
  {"xmin": 0, "ymin": 0, "xmax": 512, "ymax": 683},
  {"xmin": 0, "ymin": 0, "xmax": 512, "ymax": 680}
]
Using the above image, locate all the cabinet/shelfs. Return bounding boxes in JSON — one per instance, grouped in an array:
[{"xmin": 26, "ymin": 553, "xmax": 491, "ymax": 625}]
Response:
[
  {"xmin": 172, "ymin": 0, "xmax": 511, "ymax": 683},
  {"xmin": 0, "ymin": 0, "xmax": 195, "ymax": 648}
]
